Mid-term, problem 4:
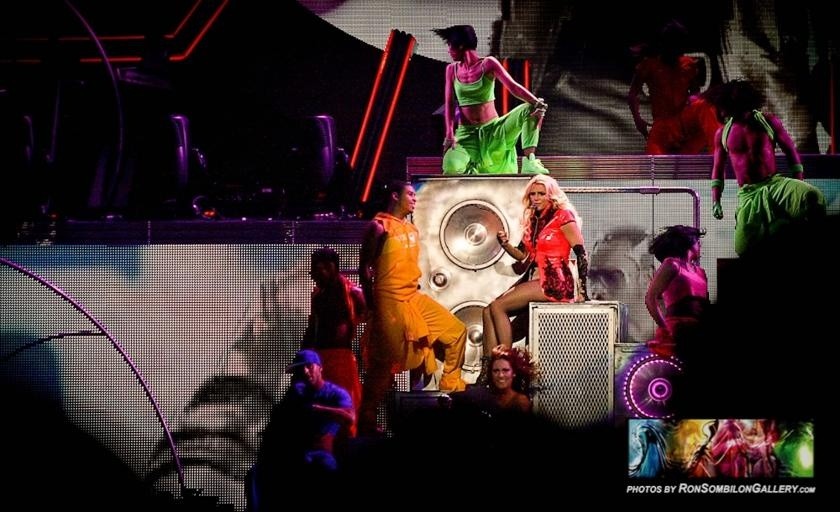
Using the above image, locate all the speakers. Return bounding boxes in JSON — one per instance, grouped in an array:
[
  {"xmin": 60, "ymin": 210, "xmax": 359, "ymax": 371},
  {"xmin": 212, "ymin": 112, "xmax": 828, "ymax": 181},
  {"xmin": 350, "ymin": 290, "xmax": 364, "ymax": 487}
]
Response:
[
  {"xmin": 614, "ymin": 341, "xmax": 686, "ymax": 425},
  {"xmin": 528, "ymin": 300, "xmax": 628, "ymax": 427},
  {"xmin": 407, "ymin": 175, "xmax": 533, "ymax": 392}
]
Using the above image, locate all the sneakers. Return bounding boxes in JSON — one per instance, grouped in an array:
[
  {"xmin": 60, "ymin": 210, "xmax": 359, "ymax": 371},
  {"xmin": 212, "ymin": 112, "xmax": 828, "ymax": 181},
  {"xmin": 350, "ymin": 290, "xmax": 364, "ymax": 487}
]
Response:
[
  {"xmin": 520, "ymin": 152, "xmax": 550, "ymax": 174},
  {"xmin": 439, "ymin": 379, "xmax": 466, "ymax": 392}
]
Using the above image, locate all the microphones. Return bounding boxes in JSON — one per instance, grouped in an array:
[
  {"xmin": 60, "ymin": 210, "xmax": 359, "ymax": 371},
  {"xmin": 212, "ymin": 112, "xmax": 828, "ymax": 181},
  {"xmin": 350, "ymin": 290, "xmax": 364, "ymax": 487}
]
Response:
[{"xmin": 533, "ymin": 204, "xmax": 538, "ymax": 209}]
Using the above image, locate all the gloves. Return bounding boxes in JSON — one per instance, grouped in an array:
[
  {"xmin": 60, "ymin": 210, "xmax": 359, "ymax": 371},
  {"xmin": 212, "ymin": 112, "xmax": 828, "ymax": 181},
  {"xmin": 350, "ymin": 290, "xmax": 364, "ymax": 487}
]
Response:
[{"xmin": 573, "ymin": 244, "xmax": 591, "ymax": 301}]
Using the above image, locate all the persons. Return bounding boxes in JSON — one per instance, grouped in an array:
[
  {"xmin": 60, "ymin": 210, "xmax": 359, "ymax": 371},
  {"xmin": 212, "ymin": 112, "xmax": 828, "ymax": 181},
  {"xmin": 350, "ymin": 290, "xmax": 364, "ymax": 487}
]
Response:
[
  {"xmin": 430, "ymin": 25, "xmax": 552, "ymax": 175},
  {"xmin": 645, "ymin": 225, "xmax": 710, "ymax": 338},
  {"xmin": 245, "ymin": 348, "xmax": 356, "ymax": 512},
  {"xmin": 628, "ymin": 34, "xmax": 726, "ymax": 155},
  {"xmin": 296, "ymin": 248, "xmax": 367, "ymax": 349},
  {"xmin": 479, "ymin": 175, "xmax": 589, "ymax": 360},
  {"xmin": 465, "ymin": 342, "xmax": 544, "ymax": 415},
  {"xmin": 710, "ymin": 79, "xmax": 826, "ymax": 262},
  {"xmin": 357, "ymin": 179, "xmax": 467, "ymax": 434}
]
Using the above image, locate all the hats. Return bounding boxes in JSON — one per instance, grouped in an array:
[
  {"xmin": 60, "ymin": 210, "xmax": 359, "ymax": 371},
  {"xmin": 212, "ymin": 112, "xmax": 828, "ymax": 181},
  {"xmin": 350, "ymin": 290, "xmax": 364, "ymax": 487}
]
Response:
[{"xmin": 285, "ymin": 350, "xmax": 321, "ymax": 373}]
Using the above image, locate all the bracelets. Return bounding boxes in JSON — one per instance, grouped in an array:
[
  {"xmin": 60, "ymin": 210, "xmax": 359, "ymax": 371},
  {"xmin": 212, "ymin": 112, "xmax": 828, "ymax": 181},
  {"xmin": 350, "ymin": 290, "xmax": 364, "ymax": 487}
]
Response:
[
  {"xmin": 710, "ymin": 179, "xmax": 724, "ymax": 192},
  {"xmin": 535, "ymin": 97, "xmax": 545, "ymax": 108},
  {"xmin": 791, "ymin": 164, "xmax": 804, "ymax": 173}
]
